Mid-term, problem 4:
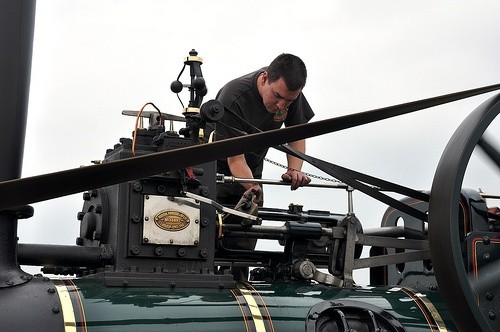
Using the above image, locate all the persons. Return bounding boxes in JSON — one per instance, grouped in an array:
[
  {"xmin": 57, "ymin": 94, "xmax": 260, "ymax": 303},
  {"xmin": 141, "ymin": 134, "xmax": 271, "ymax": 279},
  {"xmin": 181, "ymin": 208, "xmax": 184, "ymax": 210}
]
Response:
[{"xmin": 198, "ymin": 51, "xmax": 317, "ymax": 282}]
